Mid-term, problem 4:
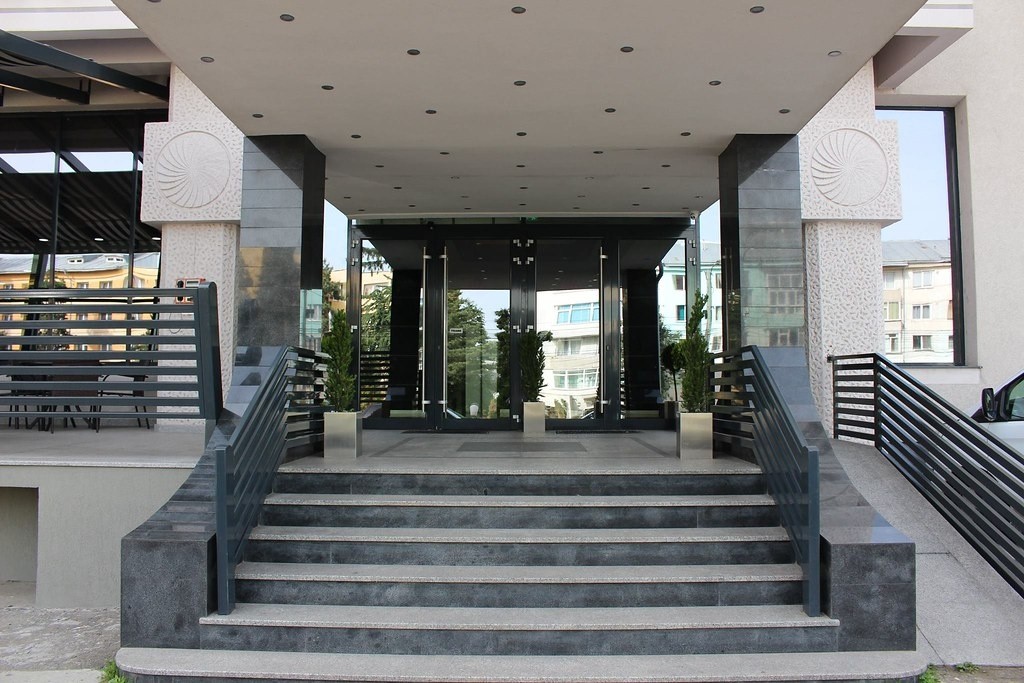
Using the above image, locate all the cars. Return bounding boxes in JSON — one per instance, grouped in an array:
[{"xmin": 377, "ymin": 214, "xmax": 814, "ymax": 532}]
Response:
[
  {"xmin": 938, "ymin": 370, "xmax": 1024, "ymax": 457},
  {"xmin": 363, "ymin": 401, "xmax": 465, "ymax": 419}
]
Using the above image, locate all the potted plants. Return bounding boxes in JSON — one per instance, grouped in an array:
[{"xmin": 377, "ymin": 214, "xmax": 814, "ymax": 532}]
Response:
[
  {"xmin": 320, "ymin": 308, "xmax": 362, "ymax": 459},
  {"xmin": 518, "ymin": 328, "xmax": 546, "ymax": 432},
  {"xmin": 671, "ymin": 287, "xmax": 714, "ymax": 460}
]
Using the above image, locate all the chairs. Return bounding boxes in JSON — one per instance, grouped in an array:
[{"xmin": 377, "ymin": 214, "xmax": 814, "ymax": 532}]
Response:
[{"xmin": 6, "ymin": 359, "xmax": 150, "ymax": 434}]
